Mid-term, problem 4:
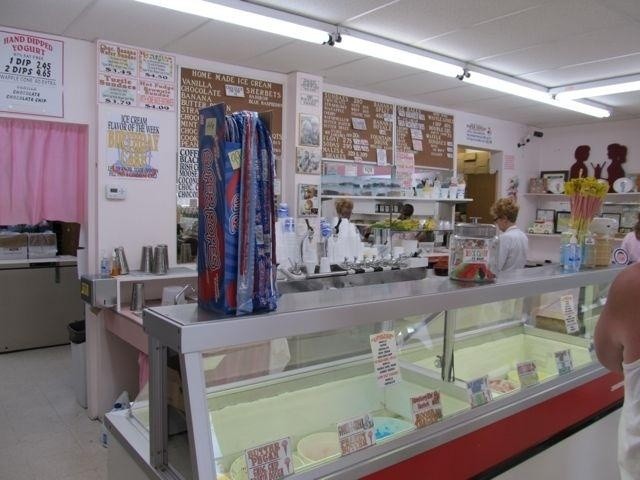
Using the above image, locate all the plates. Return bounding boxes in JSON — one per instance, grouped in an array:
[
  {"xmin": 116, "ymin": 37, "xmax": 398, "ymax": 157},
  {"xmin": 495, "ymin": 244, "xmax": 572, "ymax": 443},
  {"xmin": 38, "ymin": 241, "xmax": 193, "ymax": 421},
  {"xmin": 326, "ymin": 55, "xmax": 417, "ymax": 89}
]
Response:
[
  {"xmin": 297, "ymin": 432, "xmax": 341, "ymax": 462},
  {"xmin": 614, "ymin": 248, "xmax": 629, "ymax": 266},
  {"xmin": 228, "ymin": 453, "xmax": 304, "ymax": 478},
  {"xmin": 612, "ymin": 178, "xmax": 632, "ymax": 193},
  {"xmin": 547, "ymin": 177, "xmax": 566, "ymax": 193},
  {"xmin": 372, "ymin": 417, "xmax": 412, "ymax": 440},
  {"xmin": 594, "ymin": 178, "xmax": 610, "ymax": 192}
]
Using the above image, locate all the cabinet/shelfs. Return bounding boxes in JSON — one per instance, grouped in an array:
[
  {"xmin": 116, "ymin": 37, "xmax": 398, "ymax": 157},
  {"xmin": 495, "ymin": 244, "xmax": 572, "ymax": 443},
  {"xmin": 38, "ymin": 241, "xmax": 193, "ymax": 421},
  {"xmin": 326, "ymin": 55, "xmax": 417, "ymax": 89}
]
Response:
[
  {"xmin": 525, "ymin": 232, "xmax": 569, "ymax": 264},
  {"xmin": 390, "ymin": 195, "xmax": 475, "ymax": 256},
  {"xmin": 318, "ymin": 193, "xmax": 393, "ymax": 256},
  {"xmin": 1, "ymin": 254, "xmax": 85, "ymax": 357},
  {"xmin": 138, "ymin": 257, "xmax": 633, "ymax": 480}
]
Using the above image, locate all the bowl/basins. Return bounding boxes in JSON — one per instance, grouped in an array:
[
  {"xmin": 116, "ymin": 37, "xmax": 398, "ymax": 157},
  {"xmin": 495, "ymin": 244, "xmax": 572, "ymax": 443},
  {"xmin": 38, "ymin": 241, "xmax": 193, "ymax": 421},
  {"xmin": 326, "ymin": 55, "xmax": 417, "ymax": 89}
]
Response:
[{"xmin": 507, "ymin": 370, "xmax": 520, "ymax": 389}]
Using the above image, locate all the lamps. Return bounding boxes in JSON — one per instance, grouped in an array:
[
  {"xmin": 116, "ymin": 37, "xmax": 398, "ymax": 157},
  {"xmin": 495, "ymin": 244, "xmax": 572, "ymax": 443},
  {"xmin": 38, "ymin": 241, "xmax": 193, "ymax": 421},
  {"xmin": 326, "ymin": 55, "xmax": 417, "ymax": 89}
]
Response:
[
  {"xmin": 460, "ymin": 60, "xmax": 612, "ymax": 123},
  {"xmin": 544, "ymin": 73, "xmax": 639, "ymax": 102},
  {"xmin": 128, "ymin": 0, "xmax": 338, "ymax": 46},
  {"xmin": 332, "ymin": 24, "xmax": 467, "ymax": 81}
]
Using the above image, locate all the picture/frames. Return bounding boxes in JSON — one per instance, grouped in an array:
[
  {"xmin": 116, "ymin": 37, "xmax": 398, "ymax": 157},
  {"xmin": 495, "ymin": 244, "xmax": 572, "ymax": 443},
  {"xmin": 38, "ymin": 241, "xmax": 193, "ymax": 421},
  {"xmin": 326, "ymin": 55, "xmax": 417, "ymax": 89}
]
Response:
[
  {"xmin": 555, "ymin": 209, "xmax": 573, "ymax": 234},
  {"xmin": 602, "ymin": 212, "xmax": 623, "ymax": 234},
  {"xmin": 535, "ymin": 207, "xmax": 557, "ymax": 231},
  {"xmin": 539, "ymin": 169, "xmax": 568, "ymax": 194}
]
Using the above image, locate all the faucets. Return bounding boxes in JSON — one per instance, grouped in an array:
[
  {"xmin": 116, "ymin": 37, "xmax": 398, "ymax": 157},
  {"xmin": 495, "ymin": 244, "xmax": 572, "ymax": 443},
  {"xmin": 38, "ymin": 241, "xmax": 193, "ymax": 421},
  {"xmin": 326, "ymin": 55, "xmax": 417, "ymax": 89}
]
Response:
[{"xmin": 299, "ymin": 227, "xmax": 314, "ymax": 262}]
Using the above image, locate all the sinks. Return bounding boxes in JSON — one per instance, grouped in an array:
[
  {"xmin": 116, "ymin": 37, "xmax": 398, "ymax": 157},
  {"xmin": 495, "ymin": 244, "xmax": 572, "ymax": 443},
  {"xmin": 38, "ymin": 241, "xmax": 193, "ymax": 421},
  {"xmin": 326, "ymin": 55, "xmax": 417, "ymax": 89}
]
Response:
[{"xmin": 300, "ymin": 264, "xmax": 347, "ymax": 278}]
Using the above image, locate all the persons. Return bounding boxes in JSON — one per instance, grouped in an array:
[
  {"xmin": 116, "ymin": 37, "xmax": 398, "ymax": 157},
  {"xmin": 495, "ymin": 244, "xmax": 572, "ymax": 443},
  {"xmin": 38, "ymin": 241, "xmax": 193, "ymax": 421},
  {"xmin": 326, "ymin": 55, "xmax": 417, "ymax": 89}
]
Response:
[
  {"xmin": 299, "ymin": 118, "xmax": 318, "ymax": 172},
  {"xmin": 301, "ymin": 184, "xmax": 318, "ymax": 214},
  {"xmin": 620, "ymin": 212, "xmax": 640, "ymax": 263},
  {"xmin": 595, "ymin": 259, "xmax": 640, "ymax": 480},
  {"xmin": 487, "ymin": 199, "xmax": 528, "ymax": 271},
  {"xmin": 387, "ymin": 202, "xmax": 416, "ymax": 245},
  {"xmin": 328, "ymin": 199, "xmax": 363, "ymax": 261}
]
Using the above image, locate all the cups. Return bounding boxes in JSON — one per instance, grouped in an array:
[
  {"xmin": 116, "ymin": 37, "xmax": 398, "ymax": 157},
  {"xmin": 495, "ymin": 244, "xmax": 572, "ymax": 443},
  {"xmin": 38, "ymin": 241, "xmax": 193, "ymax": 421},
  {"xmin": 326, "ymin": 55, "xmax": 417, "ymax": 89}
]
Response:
[
  {"xmin": 304, "ymin": 260, "xmax": 316, "ymax": 274},
  {"xmin": 375, "ymin": 203, "xmax": 399, "ymax": 213},
  {"xmin": 402, "ymin": 177, "xmax": 465, "ymax": 199}
]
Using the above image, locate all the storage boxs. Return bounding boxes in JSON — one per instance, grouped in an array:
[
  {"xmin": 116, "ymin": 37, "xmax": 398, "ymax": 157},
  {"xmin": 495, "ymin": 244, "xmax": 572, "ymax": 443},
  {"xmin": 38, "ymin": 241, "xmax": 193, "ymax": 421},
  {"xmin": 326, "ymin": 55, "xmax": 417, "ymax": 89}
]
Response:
[
  {"xmin": 1, "ymin": 232, "xmax": 29, "ymax": 264},
  {"xmin": 25, "ymin": 232, "xmax": 59, "ymax": 260},
  {"xmin": 457, "ymin": 149, "xmax": 493, "ymax": 176}
]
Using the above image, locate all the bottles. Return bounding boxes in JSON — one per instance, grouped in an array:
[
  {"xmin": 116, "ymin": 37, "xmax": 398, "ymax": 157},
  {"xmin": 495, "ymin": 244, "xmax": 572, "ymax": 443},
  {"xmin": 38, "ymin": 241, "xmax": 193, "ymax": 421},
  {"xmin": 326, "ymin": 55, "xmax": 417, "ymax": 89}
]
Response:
[
  {"xmin": 111, "ymin": 251, "xmax": 119, "ymax": 276},
  {"xmin": 559, "ymin": 232, "xmax": 596, "ymax": 272},
  {"xmin": 320, "ymin": 256, "xmax": 330, "ymax": 272},
  {"xmin": 102, "ymin": 402, "xmax": 122, "ymax": 447},
  {"xmin": 129, "ymin": 282, "xmax": 145, "ymax": 312},
  {"xmin": 141, "ymin": 242, "xmax": 169, "ymax": 275},
  {"xmin": 449, "ymin": 216, "xmax": 499, "ymax": 284},
  {"xmin": 114, "ymin": 247, "xmax": 129, "ymax": 274},
  {"xmin": 180, "ymin": 243, "xmax": 192, "ymax": 262}
]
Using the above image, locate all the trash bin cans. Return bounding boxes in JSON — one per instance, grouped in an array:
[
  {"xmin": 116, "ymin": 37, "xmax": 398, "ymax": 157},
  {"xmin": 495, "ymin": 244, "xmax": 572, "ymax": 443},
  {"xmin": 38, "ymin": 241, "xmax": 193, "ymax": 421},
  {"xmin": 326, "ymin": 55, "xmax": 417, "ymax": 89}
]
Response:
[{"xmin": 67, "ymin": 321, "xmax": 87, "ymax": 409}]
